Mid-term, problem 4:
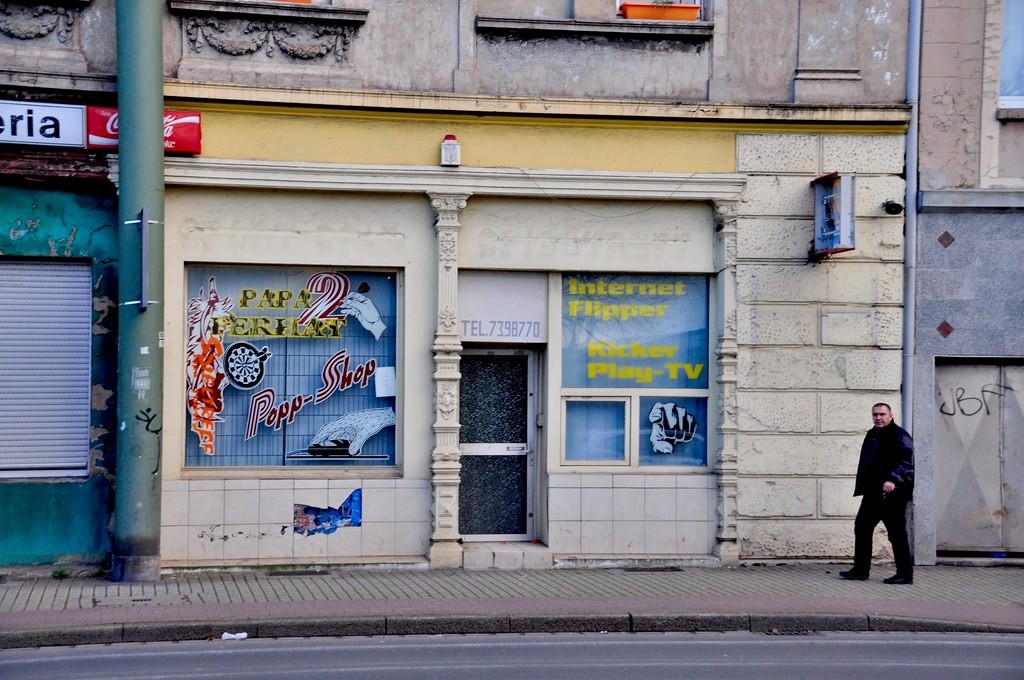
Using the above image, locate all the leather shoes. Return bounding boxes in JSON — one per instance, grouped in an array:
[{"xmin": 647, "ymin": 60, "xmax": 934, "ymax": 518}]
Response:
[
  {"xmin": 839, "ymin": 567, "xmax": 869, "ymax": 580},
  {"xmin": 883, "ymin": 574, "xmax": 913, "ymax": 584}
]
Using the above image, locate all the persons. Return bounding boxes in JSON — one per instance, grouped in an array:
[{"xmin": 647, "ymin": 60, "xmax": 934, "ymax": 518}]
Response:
[{"xmin": 839, "ymin": 403, "xmax": 915, "ymax": 584}]
